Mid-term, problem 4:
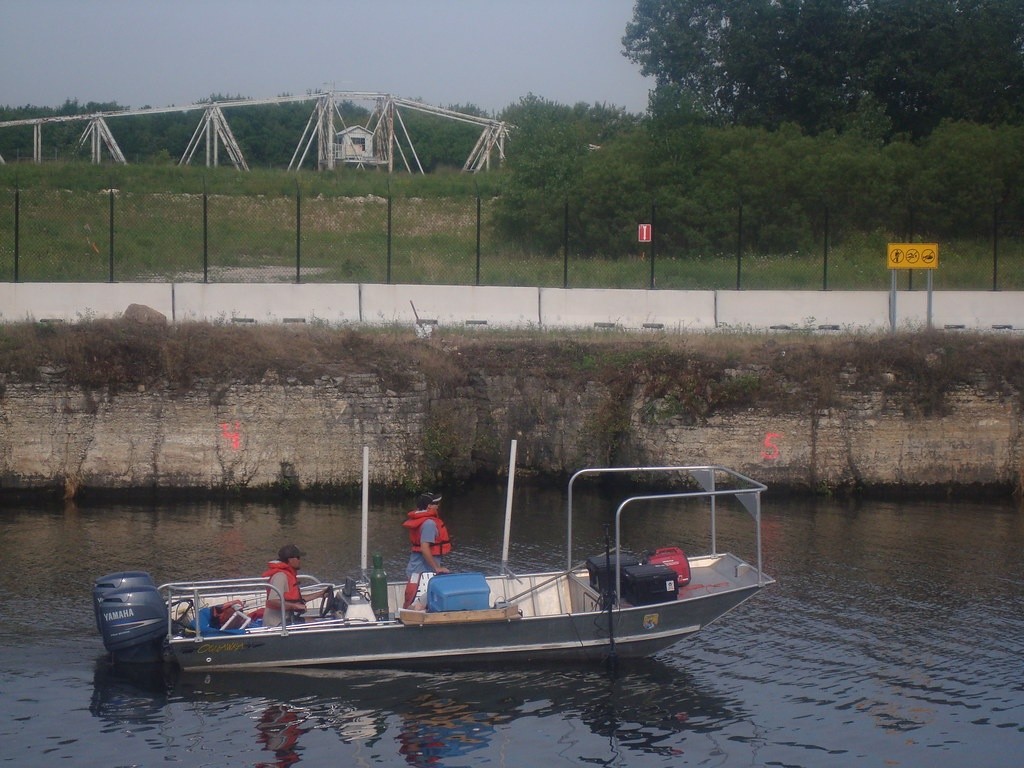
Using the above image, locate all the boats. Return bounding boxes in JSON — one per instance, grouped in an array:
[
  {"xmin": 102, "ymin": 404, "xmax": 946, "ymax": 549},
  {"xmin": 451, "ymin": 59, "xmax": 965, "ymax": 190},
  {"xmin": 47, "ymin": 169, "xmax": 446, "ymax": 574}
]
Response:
[{"xmin": 90, "ymin": 430, "xmax": 777, "ymax": 677}]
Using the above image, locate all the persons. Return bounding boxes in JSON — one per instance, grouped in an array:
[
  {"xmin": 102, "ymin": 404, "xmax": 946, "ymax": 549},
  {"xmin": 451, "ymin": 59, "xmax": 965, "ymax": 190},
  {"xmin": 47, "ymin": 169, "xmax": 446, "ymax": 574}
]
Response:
[
  {"xmin": 262, "ymin": 544, "xmax": 328, "ymax": 627},
  {"xmin": 402, "ymin": 491, "xmax": 452, "ymax": 609}
]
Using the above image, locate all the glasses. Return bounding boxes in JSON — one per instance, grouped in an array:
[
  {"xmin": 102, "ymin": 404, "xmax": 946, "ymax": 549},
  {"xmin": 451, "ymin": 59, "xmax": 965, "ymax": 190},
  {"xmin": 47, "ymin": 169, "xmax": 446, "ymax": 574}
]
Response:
[
  {"xmin": 290, "ymin": 556, "xmax": 299, "ymax": 559},
  {"xmin": 431, "ymin": 501, "xmax": 438, "ymax": 505}
]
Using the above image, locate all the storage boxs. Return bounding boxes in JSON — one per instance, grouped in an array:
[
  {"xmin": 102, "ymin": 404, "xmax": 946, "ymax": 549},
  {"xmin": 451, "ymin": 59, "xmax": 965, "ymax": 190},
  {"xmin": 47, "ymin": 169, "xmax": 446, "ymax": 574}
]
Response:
[
  {"xmin": 425, "ymin": 571, "xmax": 492, "ymax": 613},
  {"xmin": 622, "ymin": 564, "xmax": 680, "ymax": 607},
  {"xmin": 585, "ymin": 552, "xmax": 639, "ymax": 600}
]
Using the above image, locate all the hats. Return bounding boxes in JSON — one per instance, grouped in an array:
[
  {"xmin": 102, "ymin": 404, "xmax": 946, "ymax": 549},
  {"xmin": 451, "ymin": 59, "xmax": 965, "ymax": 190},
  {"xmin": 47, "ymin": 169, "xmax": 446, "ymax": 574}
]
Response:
[
  {"xmin": 417, "ymin": 492, "xmax": 442, "ymax": 506},
  {"xmin": 278, "ymin": 545, "xmax": 306, "ymax": 559}
]
